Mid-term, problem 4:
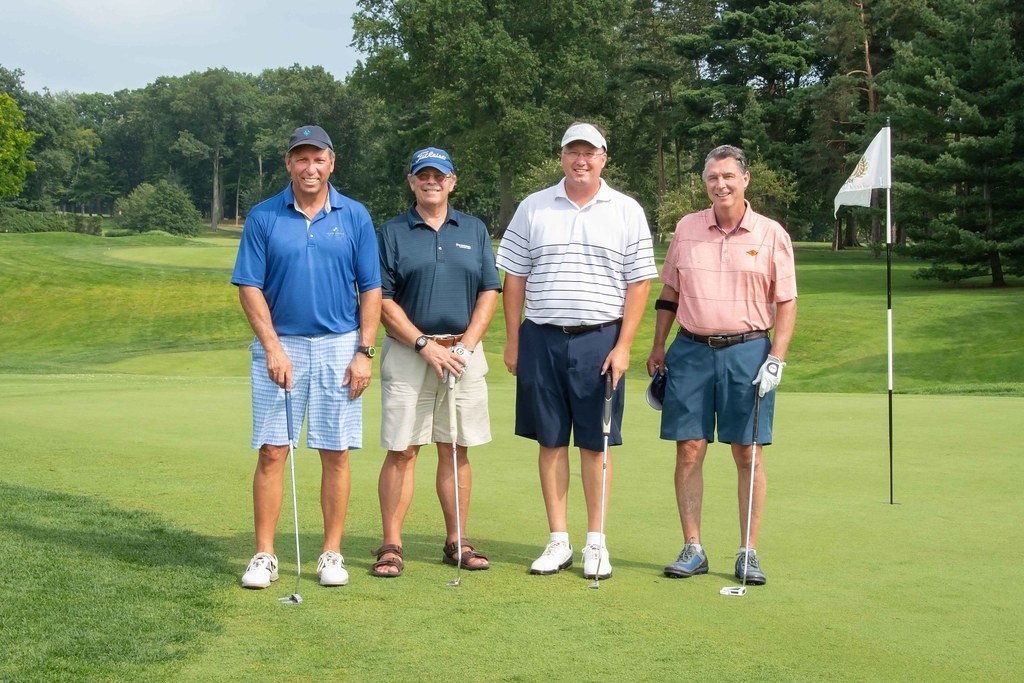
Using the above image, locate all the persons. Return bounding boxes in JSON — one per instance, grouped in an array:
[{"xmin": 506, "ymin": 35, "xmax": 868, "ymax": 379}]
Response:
[
  {"xmin": 370, "ymin": 147, "xmax": 517, "ymax": 576},
  {"xmin": 496, "ymin": 121, "xmax": 660, "ymax": 580},
  {"xmin": 230, "ymin": 125, "xmax": 382, "ymax": 589},
  {"xmin": 646, "ymin": 145, "xmax": 797, "ymax": 584}
]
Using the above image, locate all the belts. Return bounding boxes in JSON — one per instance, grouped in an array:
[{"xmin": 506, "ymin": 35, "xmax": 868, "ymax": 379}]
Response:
[
  {"xmin": 385, "ymin": 332, "xmax": 463, "ymax": 348},
  {"xmin": 678, "ymin": 327, "xmax": 768, "ymax": 348},
  {"xmin": 546, "ymin": 318, "xmax": 621, "ymax": 333}
]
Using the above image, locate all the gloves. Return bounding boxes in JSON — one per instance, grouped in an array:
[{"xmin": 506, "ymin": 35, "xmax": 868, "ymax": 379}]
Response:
[
  {"xmin": 752, "ymin": 354, "xmax": 786, "ymax": 396},
  {"xmin": 443, "ymin": 346, "xmax": 473, "ymax": 388}
]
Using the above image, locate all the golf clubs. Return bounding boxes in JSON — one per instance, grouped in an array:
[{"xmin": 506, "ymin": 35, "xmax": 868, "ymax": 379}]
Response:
[
  {"xmin": 443, "ymin": 384, "xmax": 461, "ymax": 586},
  {"xmin": 587, "ymin": 370, "xmax": 613, "ymax": 590},
  {"xmin": 719, "ymin": 382, "xmax": 761, "ymax": 597},
  {"xmin": 277, "ymin": 391, "xmax": 303, "ymax": 605}
]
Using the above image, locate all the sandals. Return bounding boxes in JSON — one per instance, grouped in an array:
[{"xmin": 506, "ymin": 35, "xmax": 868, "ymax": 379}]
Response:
[
  {"xmin": 442, "ymin": 538, "xmax": 489, "ymax": 570},
  {"xmin": 371, "ymin": 544, "xmax": 403, "ymax": 577}
]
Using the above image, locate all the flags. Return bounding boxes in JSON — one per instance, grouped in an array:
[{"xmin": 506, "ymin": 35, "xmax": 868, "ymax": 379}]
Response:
[{"xmin": 834, "ymin": 127, "xmax": 891, "ymax": 219}]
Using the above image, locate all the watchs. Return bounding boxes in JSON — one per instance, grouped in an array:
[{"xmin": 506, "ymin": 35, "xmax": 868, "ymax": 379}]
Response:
[
  {"xmin": 357, "ymin": 346, "xmax": 376, "ymax": 358},
  {"xmin": 415, "ymin": 336, "xmax": 429, "ymax": 353}
]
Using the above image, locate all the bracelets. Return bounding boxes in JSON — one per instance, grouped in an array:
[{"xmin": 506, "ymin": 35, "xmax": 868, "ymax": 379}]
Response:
[{"xmin": 655, "ymin": 299, "xmax": 678, "ymax": 314}]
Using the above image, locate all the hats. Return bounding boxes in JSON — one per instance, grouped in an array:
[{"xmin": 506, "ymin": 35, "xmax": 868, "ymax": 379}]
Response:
[
  {"xmin": 410, "ymin": 147, "xmax": 454, "ymax": 175},
  {"xmin": 561, "ymin": 123, "xmax": 607, "ymax": 149},
  {"xmin": 288, "ymin": 126, "xmax": 333, "ymax": 153},
  {"xmin": 647, "ymin": 365, "xmax": 669, "ymax": 412}
]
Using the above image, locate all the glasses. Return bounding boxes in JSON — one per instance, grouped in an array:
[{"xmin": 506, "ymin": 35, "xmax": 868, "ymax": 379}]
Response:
[
  {"xmin": 415, "ymin": 171, "xmax": 451, "ymax": 182},
  {"xmin": 561, "ymin": 150, "xmax": 606, "ymax": 160}
]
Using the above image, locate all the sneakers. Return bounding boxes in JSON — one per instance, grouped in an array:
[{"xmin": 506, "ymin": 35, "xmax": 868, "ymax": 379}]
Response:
[
  {"xmin": 530, "ymin": 542, "xmax": 574, "ymax": 575},
  {"xmin": 242, "ymin": 552, "xmax": 279, "ymax": 589},
  {"xmin": 581, "ymin": 543, "xmax": 612, "ymax": 579},
  {"xmin": 317, "ymin": 550, "xmax": 349, "ymax": 585},
  {"xmin": 664, "ymin": 537, "xmax": 709, "ymax": 577},
  {"xmin": 735, "ymin": 550, "xmax": 766, "ymax": 584}
]
